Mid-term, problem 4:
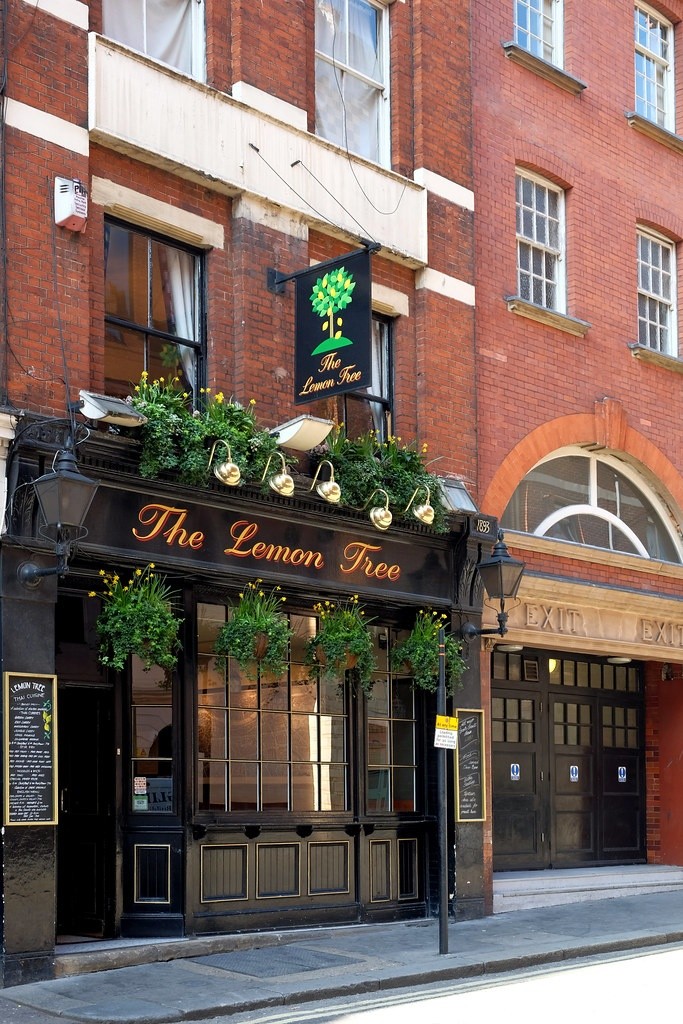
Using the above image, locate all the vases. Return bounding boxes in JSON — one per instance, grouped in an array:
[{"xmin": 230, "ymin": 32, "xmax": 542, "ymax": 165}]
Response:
[
  {"xmin": 252, "ymin": 631, "xmax": 270, "ymax": 659},
  {"xmin": 314, "ymin": 643, "xmax": 358, "ymax": 670},
  {"xmin": 405, "ymin": 659, "xmax": 448, "ymax": 674}
]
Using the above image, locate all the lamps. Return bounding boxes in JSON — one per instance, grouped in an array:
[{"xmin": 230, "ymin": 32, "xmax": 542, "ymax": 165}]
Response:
[
  {"xmin": 204, "ymin": 440, "xmax": 242, "ymax": 487},
  {"xmin": 334, "ymin": 489, "xmax": 393, "ymax": 532},
  {"xmin": 16, "ymin": 436, "xmax": 101, "ymax": 589},
  {"xmin": 393, "ymin": 485, "xmax": 434, "ymax": 526},
  {"xmin": 606, "ymin": 656, "xmax": 631, "ymax": 665},
  {"xmin": 496, "ymin": 644, "xmax": 524, "ymax": 652},
  {"xmin": 460, "ymin": 529, "xmax": 527, "ymax": 644},
  {"xmin": 68, "ymin": 390, "xmax": 148, "ymax": 429},
  {"xmin": 296, "ymin": 460, "xmax": 341, "ymax": 504},
  {"xmin": 259, "ymin": 450, "xmax": 295, "ymax": 497},
  {"xmin": 268, "ymin": 413, "xmax": 335, "ymax": 453},
  {"xmin": 437, "ymin": 476, "xmax": 479, "ymax": 514}
]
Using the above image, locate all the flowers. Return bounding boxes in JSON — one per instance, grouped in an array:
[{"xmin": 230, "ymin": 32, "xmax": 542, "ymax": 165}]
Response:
[
  {"xmin": 128, "ymin": 370, "xmax": 451, "ymax": 535},
  {"xmin": 302, "ymin": 595, "xmax": 378, "ymax": 703},
  {"xmin": 86, "ymin": 562, "xmax": 183, "ymax": 674},
  {"xmin": 213, "ymin": 578, "xmax": 294, "ymax": 686},
  {"xmin": 389, "ymin": 608, "xmax": 470, "ymax": 699}
]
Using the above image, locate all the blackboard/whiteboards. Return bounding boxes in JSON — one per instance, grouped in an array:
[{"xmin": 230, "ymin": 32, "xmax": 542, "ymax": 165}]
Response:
[
  {"xmin": 455, "ymin": 708, "xmax": 487, "ymax": 823},
  {"xmin": 3, "ymin": 671, "xmax": 59, "ymax": 825}
]
